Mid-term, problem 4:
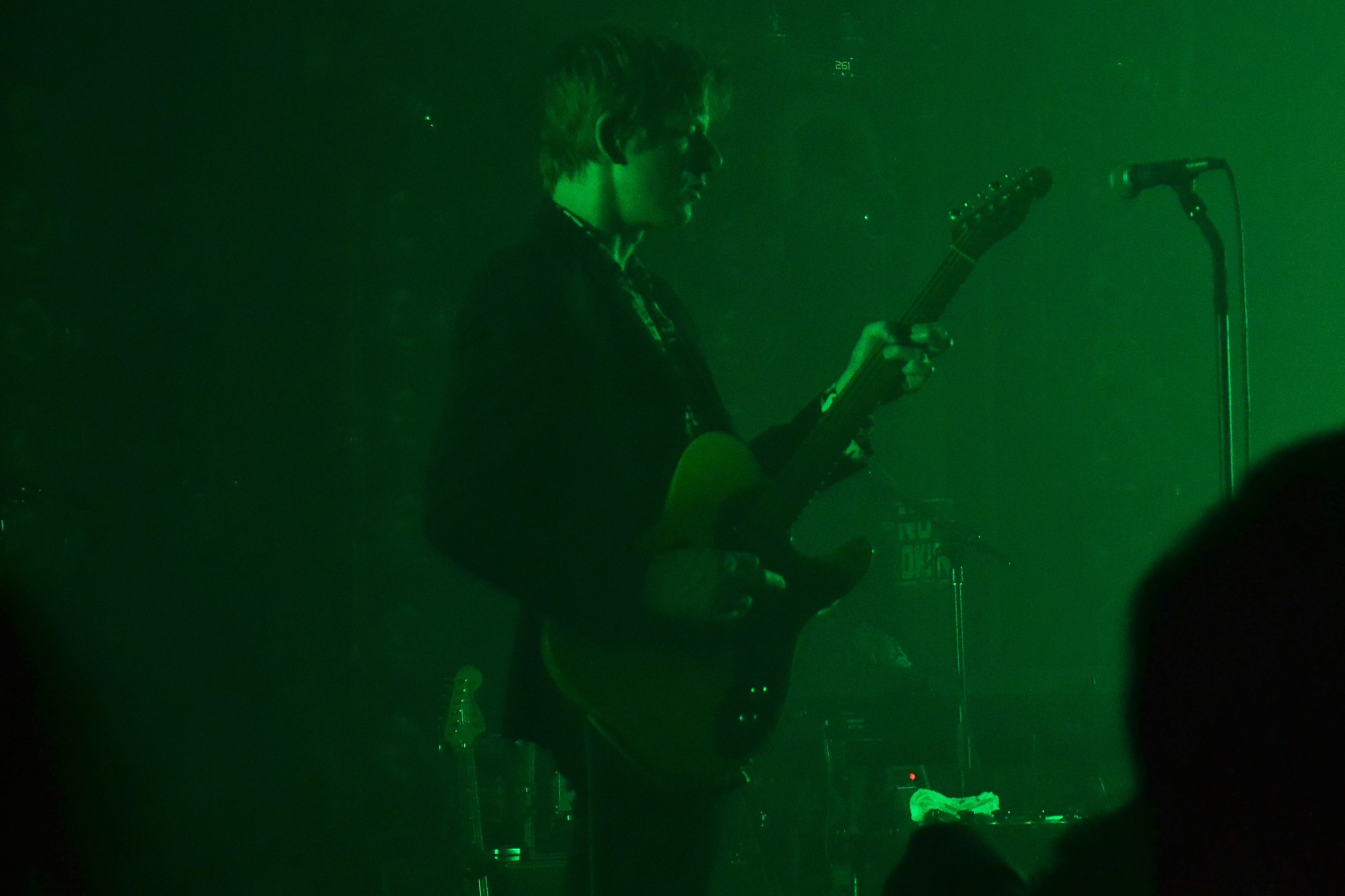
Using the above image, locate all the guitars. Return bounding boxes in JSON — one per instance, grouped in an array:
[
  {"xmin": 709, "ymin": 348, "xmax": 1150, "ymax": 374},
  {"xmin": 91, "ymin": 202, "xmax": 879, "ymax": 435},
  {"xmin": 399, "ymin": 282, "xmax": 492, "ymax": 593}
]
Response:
[{"xmin": 542, "ymin": 164, "xmax": 1055, "ymax": 781}]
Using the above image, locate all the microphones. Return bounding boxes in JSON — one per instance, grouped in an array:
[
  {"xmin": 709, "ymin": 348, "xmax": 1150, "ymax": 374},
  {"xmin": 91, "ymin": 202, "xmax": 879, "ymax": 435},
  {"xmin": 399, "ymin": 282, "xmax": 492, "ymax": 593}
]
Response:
[
  {"xmin": 1112, "ymin": 156, "xmax": 1227, "ymax": 198},
  {"xmin": 438, "ymin": 664, "xmax": 484, "ymax": 755}
]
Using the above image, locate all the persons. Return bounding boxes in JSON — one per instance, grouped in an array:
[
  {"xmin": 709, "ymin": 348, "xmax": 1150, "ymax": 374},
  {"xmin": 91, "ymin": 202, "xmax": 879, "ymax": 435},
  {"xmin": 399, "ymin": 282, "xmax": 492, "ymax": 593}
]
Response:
[
  {"xmin": 420, "ymin": 19, "xmax": 956, "ymax": 895},
  {"xmin": 872, "ymin": 423, "xmax": 1344, "ymax": 896}
]
